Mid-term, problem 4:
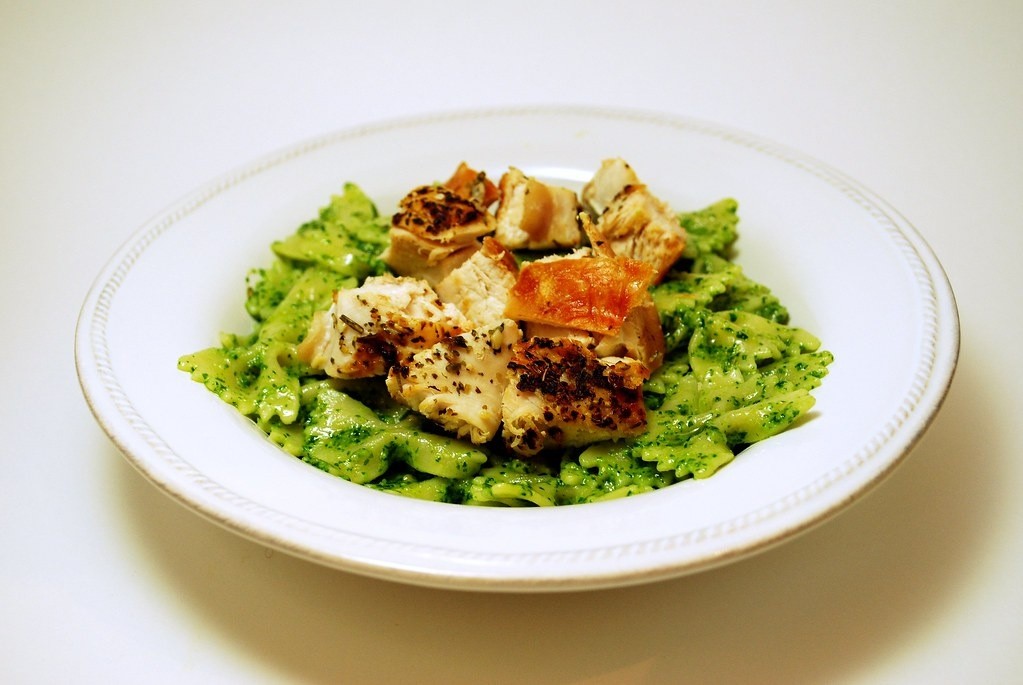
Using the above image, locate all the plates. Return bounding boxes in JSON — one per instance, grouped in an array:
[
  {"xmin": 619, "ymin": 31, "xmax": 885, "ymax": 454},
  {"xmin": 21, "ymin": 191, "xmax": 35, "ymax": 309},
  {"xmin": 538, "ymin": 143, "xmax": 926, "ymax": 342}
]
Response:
[{"xmin": 74, "ymin": 105, "xmax": 961, "ymax": 593}]
